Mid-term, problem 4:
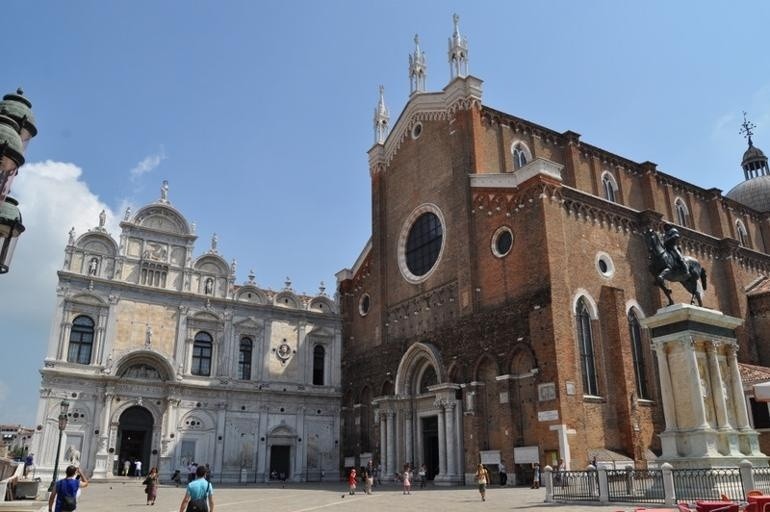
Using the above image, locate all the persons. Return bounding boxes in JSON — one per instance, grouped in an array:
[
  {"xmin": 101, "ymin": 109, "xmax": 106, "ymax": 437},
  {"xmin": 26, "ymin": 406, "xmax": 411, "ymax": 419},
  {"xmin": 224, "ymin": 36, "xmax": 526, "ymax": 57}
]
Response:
[
  {"xmin": 556, "ymin": 458, "xmax": 565, "ymax": 483},
  {"xmin": 662, "ymin": 221, "xmax": 691, "ymax": 275},
  {"xmin": 172, "ymin": 460, "xmax": 211, "ymax": 489},
  {"xmin": 474, "ymin": 463, "xmax": 490, "ymax": 502},
  {"xmin": 531, "ymin": 461, "xmax": 541, "ymax": 489},
  {"xmin": 179, "ymin": 466, "xmax": 216, "ymax": 512},
  {"xmin": 48, "ymin": 465, "xmax": 88, "ymax": 512},
  {"xmin": 134, "ymin": 458, "xmax": 142, "ymax": 480},
  {"xmin": 124, "ymin": 459, "xmax": 131, "ymax": 476},
  {"xmin": 346, "ymin": 459, "xmax": 428, "ymax": 495},
  {"xmin": 145, "ymin": 466, "xmax": 160, "ymax": 506},
  {"xmin": 280, "ymin": 470, "xmax": 286, "ymax": 488},
  {"xmin": 25, "ymin": 453, "xmax": 34, "ymax": 480},
  {"xmin": 497, "ymin": 459, "xmax": 507, "ymax": 488}
]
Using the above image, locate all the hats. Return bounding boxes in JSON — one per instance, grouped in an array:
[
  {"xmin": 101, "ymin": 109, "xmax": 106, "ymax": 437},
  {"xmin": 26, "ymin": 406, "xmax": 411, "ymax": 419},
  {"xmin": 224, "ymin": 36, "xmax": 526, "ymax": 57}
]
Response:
[
  {"xmin": 196, "ymin": 466, "xmax": 207, "ymax": 472},
  {"xmin": 477, "ymin": 464, "xmax": 483, "ymax": 467}
]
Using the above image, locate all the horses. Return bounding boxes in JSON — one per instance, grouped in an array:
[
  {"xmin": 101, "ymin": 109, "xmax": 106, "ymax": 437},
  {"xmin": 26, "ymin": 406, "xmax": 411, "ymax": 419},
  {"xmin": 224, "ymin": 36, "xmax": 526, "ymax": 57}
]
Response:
[{"xmin": 643, "ymin": 229, "xmax": 707, "ymax": 305}]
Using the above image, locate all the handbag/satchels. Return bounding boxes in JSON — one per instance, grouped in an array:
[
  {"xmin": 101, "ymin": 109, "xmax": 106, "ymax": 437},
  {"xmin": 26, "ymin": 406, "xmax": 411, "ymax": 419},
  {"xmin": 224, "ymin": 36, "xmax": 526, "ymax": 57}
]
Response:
[
  {"xmin": 145, "ymin": 485, "xmax": 151, "ymax": 493},
  {"xmin": 188, "ymin": 499, "xmax": 208, "ymax": 512},
  {"xmin": 61, "ymin": 495, "xmax": 76, "ymax": 511}
]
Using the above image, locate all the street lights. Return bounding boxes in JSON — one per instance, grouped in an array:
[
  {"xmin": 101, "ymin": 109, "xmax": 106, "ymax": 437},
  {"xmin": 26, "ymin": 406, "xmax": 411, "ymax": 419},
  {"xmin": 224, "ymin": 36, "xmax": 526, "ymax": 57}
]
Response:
[
  {"xmin": 47, "ymin": 399, "xmax": 70, "ymax": 501},
  {"xmin": 19, "ymin": 435, "xmax": 28, "ymax": 461},
  {"xmin": 0, "ymin": 80, "xmax": 40, "ymax": 284}
]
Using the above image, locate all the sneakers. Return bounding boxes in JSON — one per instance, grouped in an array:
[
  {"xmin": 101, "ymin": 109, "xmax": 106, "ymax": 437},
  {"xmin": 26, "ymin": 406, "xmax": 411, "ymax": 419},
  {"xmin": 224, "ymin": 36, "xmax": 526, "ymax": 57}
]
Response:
[
  {"xmin": 146, "ymin": 502, "xmax": 155, "ymax": 505},
  {"xmin": 481, "ymin": 496, "xmax": 485, "ymax": 502}
]
